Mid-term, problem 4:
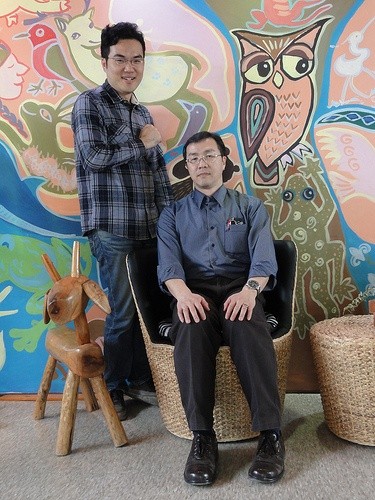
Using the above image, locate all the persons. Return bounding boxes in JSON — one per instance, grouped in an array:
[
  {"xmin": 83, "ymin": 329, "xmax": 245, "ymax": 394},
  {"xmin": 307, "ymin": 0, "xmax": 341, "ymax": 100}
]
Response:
[
  {"xmin": 156, "ymin": 130, "xmax": 284, "ymax": 487},
  {"xmin": 70, "ymin": 21, "xmax": 174, "ymax": 422}
]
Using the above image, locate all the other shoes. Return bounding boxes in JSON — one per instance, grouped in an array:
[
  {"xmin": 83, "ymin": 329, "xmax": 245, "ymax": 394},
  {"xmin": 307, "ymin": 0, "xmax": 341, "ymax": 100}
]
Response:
[
  {"xmin": 130, "ymin": 377, "xmax": 156, "ymax": 392},
  {"xmin": 110, "ymin": 387, "xmax": 128, "ymax": 420}
]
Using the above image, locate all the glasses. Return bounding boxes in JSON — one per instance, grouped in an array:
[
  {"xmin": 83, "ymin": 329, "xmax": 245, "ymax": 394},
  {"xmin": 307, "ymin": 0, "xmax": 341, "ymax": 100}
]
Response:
[
  {"xmin": 185, "ymin": 153, "xmax": 224, "ymax": 165},
  {"xmin": 104, "ymin": 56, "xmax": 144, "ymax": 67}
]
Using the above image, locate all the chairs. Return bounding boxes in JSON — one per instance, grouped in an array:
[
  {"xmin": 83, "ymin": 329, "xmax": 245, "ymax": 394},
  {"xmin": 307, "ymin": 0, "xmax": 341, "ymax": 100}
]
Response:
[{"xmin": 128, "ymin": 239, "xmax": 298, "ymax": 442}]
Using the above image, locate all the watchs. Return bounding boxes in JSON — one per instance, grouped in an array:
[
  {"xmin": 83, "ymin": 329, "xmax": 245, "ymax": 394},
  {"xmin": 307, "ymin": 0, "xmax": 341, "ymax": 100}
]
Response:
[{"xmin": 246, "ymin": 280, "xmax": 260, "ymax": 296}]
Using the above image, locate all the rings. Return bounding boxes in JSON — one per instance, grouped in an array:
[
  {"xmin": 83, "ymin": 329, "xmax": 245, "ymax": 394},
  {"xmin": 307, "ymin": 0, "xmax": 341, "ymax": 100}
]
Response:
[{"xmin": 244, "ymin": 304, "xmax": 248, "ymax": 307}]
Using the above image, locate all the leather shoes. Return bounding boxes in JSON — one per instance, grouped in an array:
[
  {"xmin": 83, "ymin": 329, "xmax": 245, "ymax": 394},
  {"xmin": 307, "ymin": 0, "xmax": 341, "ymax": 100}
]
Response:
[
  {"xmin": 248, "ymin": 430, "xmax": 285, "ymax": 483},
  {"xmin": 184, "ymin": 431, "xmax": 218, "ymax": 486}
]
